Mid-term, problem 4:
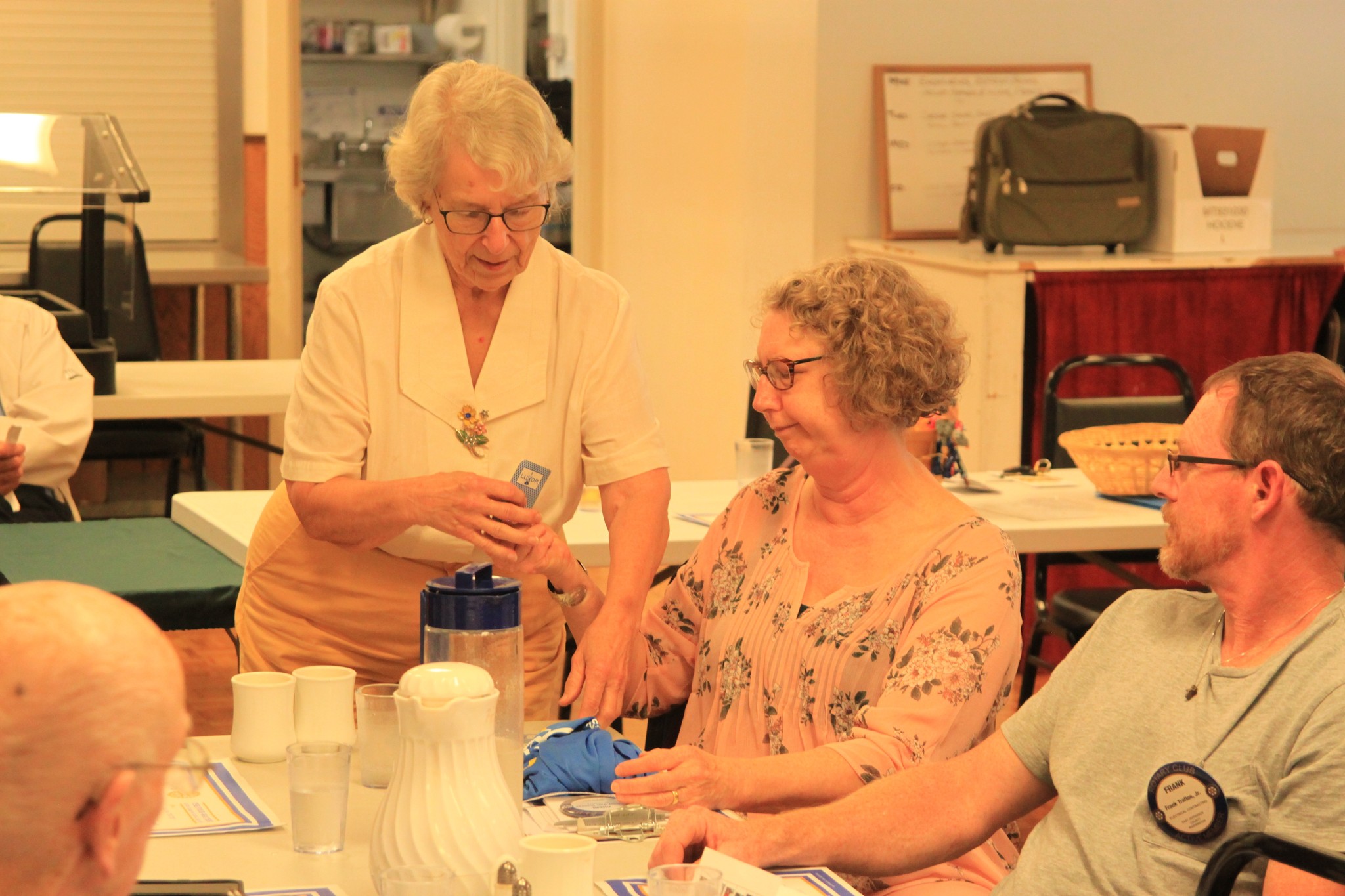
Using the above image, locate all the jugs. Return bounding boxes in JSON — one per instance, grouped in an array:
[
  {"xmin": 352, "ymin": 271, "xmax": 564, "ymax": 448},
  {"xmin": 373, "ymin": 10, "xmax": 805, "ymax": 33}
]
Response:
[
  {"xmin": 367, "ymin": 661, "xmax": 528, "ymax": 896},
  {"xmin": 418, "ymin": 561, "xmax": 524, "ymax": 821}
]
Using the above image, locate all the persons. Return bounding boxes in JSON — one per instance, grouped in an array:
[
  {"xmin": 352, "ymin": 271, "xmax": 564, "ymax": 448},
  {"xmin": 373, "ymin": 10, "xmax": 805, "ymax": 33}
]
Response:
[
  {"xmin": 647, "ymin": 350, "xmax": 1344, "ymax": 896},
  {"xmin": 481, "ymin": 258, "xmax": 1025, "ymax": 896},
  {"xmin": 0, "ymin": 293, "xmax": 94, "ymax": 524},
  {"xmin": 0, "ymin": 577, "xmax": 195, "ymax": 896},
  {"xmin": 233, "ymin": 58, "xmax": 673, "ymax": 730}
]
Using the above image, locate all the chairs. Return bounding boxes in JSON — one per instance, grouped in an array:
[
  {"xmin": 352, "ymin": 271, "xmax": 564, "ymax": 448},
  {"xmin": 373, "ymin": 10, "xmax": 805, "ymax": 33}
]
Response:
[
  {"xmin": 31, "ymin": 211, "xmax": 207, "ymax": 517},
  {"xmin": 1192, "ymin": 831, "xmax": 1345, "ymax": 896},
  {"xmin": 1014, "ymin": 352, "xmax": 1214, "ymax": 714}
]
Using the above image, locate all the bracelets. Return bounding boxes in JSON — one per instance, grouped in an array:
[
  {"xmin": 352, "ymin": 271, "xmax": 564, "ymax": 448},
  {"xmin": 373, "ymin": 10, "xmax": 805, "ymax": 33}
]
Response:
[{"xmin": 546, "ymin": 558, "xmax": 589, "ymax": 607}]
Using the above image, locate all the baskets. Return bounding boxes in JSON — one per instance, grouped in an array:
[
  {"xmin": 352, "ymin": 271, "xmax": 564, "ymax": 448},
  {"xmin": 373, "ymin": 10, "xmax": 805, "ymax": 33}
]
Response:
[{"xmin": 1061, "ymin": 421, "xmax": 1186, "ymax": 501}]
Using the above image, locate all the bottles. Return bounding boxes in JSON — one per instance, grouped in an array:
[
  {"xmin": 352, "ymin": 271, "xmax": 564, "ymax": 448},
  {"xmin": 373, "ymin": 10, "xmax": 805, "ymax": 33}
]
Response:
[{"xmin": 493, "ymin": 861, "xmax": 532, "ymax": 896}]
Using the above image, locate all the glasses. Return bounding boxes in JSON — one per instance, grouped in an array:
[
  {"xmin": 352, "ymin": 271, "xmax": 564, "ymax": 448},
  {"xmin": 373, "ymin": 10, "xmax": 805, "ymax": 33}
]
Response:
[
  {"xmin": 71, "ymin": 738, "xmax": 212, "ymax": 821},
  {"xmin": 742, "ymin": 354, "xmax": 823, "ymax": 392},
  {"xmin": 1167, "ymin": 448, "xmax": 1257, "ymax": 477},
  {"xmin": 432, "ymin": 182, "xmax": 552, "ymax": 235}
]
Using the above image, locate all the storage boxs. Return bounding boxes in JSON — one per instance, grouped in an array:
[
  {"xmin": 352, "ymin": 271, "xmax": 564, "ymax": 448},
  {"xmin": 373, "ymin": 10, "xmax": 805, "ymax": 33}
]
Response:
[{"xmin": 1140, "ymin": 122, "xmax": 1272, "ymax": 253}]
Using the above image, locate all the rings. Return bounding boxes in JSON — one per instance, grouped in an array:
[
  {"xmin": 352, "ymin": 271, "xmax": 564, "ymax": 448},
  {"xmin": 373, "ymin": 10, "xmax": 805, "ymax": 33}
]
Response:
[{"xmin": 671, "ymin": 790, "xmax": 678, "ymax": 806}]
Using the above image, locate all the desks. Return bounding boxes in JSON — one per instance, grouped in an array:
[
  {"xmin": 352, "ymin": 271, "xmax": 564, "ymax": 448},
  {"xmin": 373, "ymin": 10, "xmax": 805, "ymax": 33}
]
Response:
[{"xmin": 0, "ymin": 234, "xmax": 1345, "ymax": 896}]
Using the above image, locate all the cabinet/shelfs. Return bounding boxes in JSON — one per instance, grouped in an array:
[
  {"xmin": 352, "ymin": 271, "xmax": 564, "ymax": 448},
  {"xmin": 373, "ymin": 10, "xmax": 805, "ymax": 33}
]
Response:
[{"xmin": 299, "ymin": 0, "xmax": 458, "ymax": 307}]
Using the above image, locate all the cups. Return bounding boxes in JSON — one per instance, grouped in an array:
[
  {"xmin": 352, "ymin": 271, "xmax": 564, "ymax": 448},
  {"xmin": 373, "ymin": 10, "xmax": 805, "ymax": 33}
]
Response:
[
  {"xmin": 229, "ymin": 671, "xmax": 297, "ymax": 765},
  {"xmin": 647, "ymin": 862, "xmax": 724, "ymax": 896},
  {"xmin": 292, "ymin": 664, "xmax": 357, "ymax": 754},
  {"xmin": 735, "ymin": 438, "xmax": 776, "ymax": 493},
  {"xmin": 287, "ymin": 740, "xmax": 353, "ymax": 854},
  {"xmin": 354, "ymin": 682, "xmax": 401, "ymax": 788},
  {"xmin": 520, "ymin": 831, "xmax": 598, "ymax": 896}
]
